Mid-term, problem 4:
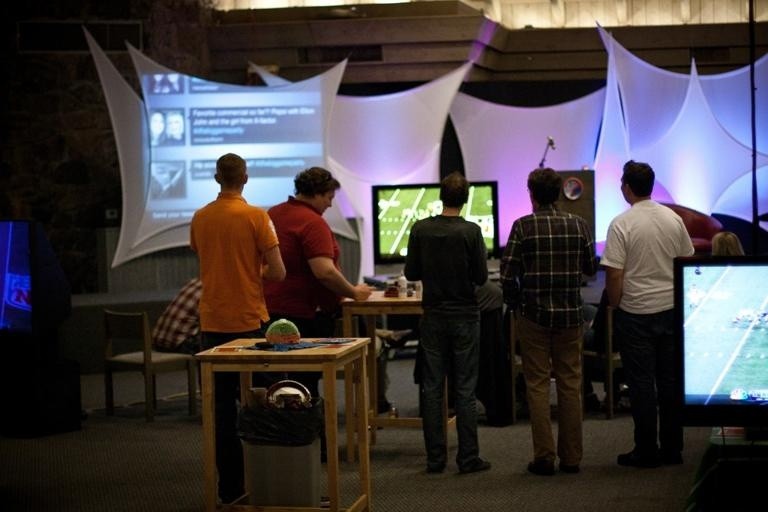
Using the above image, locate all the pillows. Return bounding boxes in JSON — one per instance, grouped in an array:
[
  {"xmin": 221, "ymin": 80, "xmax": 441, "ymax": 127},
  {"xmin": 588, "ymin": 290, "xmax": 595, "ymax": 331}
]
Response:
[{"xmin": 153, "ymin": 277, "xmax": 202, "ymax": 347}]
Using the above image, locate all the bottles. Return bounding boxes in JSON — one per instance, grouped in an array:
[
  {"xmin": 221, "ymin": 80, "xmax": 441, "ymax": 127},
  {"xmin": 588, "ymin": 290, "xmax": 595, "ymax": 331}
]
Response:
[
  {"xmin": 386, "ymin": 276, "xmax": 395, "ymax": 286},
  {"xmin": 397, "ymin": 273, "xmax": 408, "ymax": 299}
]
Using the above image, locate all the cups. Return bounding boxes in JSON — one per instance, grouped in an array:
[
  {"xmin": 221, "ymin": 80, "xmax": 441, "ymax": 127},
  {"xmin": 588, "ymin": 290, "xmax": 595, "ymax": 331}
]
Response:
[{"xmin": 245, "ymin": 386, "xmax": 268, "ymax": 406}]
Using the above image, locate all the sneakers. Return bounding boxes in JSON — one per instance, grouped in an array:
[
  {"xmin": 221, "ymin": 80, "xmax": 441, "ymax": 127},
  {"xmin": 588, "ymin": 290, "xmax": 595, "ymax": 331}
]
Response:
[
  {"xmin": 528, "ymin": 456, "xmax": 579, "ymax": 475},
  {"xmin": 460, "ymin": 462, "xmax": 490, "ymax": 473},
  {"xmin": 617, "ymin": 448, "xmax": 684, "ymax": 469}
]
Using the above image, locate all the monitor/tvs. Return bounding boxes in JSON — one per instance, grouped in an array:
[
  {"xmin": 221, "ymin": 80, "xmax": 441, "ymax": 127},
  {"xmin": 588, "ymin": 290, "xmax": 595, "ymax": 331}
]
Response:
[
  {"xmin": 371, "ymin": 180, "xmax": 499, "ymax": 265},
  {"xmin": 671, "ymin": 257, "xmax": 768, "ymax": 442}
]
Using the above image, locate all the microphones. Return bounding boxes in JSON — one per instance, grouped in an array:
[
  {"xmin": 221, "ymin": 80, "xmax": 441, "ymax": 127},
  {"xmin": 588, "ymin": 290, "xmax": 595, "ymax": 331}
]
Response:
[{"xmin": 547, "ymin": 135, "xmax": 556, "ymax": 150}]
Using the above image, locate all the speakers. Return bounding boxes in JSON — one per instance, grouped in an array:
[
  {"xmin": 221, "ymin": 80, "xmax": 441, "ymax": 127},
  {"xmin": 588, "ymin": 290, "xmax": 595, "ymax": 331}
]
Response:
[{"xmin": 556, "ymin": 170, "xmax": 594, "ymax": 256}]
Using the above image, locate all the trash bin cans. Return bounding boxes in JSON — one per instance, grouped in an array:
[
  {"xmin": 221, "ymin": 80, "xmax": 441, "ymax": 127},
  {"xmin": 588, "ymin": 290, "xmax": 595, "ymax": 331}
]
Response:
[{"xmin": 235, "ymin": 397, "xmax": 323, "ymax": 507}]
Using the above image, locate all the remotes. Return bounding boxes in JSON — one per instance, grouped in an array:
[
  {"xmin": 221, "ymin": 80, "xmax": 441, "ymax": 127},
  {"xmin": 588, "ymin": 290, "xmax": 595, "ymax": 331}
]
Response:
[{"xmin": 364, "ymin": 277, "xmax": 387, "ymax": 289}]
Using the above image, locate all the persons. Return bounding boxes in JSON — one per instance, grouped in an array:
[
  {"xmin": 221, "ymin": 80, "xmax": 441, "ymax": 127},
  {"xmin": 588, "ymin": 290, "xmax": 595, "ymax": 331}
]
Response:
[
  {"xmin": 599, "ymin": 160, "xmax": 694, "ymax": 467},
  {"xmin": 402, "ymin": 170, "xmax": 491, "ymax": 475},
  {"xmin": 260, "ymin": 165, "xmax": 377, "ymax": 396},
  {"xmin": 149, "ymin": 275, "xmax": 204, "ymax": 396},
  {"xmin": 190, "ymin": 153, "xmax": 287, "ymax": 504},
  {"xmin": 502, "ymin": 166, "xmax": 597, "ymax": 475},
  {"xmin": 711, "ymin": 232, "xmax": 747, "ymax": 256},
  {"xmin": 344, "ymin": 273, "xmax": 630, "ymax": 427}
]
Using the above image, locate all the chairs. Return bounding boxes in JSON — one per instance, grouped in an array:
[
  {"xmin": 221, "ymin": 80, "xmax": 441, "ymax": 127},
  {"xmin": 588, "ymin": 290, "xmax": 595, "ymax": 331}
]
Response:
[
  {"xmin": 502, "ymin": 306, "xmax": 532, "ymax": 423},
  {"xmin": 105, "ymin": 311, "xmax": 197, "ymax": 423},
  {"xmin": 582, "ymin": 302, "xmax": 629, "ymax": 417}
]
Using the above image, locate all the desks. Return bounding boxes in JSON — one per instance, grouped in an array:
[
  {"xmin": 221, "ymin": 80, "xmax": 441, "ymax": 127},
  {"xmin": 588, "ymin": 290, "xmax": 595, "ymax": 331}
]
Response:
[
  {"xmin": 341, "ymin": 290, "xmax": 467, "ymax": 457},
  {"xmin": 195, "ymin": 338, "xmax": 371, "ymax": 508}
]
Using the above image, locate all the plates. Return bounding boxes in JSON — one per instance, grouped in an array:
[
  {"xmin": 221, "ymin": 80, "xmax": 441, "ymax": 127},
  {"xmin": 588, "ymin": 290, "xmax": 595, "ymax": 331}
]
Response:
[{"xmin": 267, "ymin": 379, "xmax": 312, "ymax": 405}]
